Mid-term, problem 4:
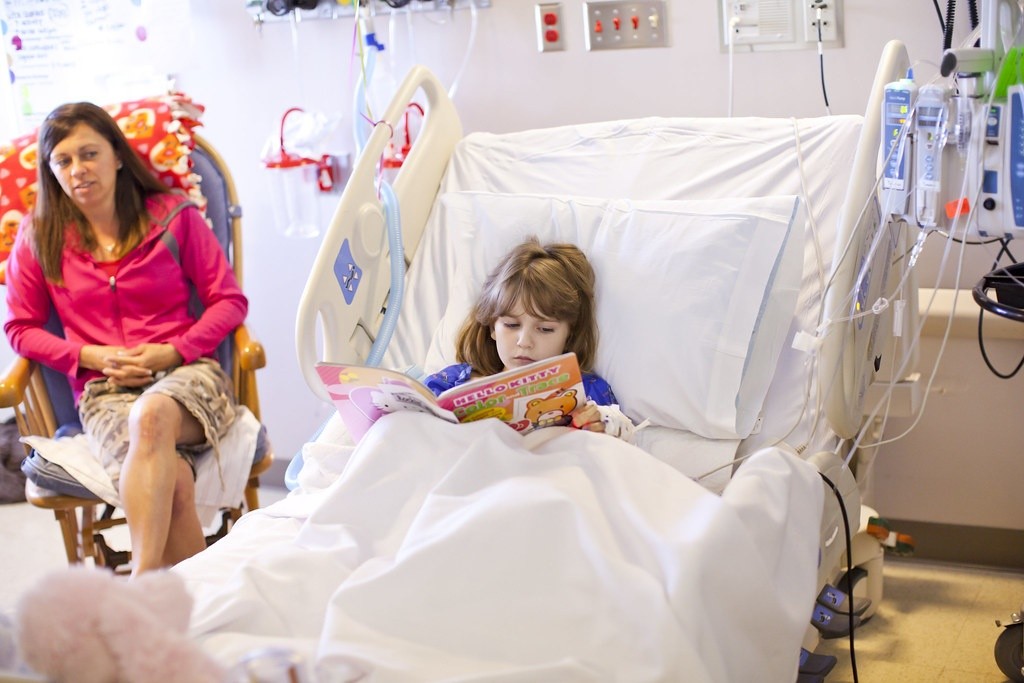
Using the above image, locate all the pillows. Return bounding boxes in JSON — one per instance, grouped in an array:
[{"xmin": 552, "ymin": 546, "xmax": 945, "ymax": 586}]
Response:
[
  {"xmin": 421, "ymin": 188, "xmax": 804, "ymax": 442},
  {"xmin": 0, "ymin": 91, "xmax": 209, "ymax": 294}
]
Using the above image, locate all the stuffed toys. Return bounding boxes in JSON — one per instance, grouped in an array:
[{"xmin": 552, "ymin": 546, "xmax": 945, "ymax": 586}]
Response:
[{"xmin": 0, "ymin": 566, "xmax": 221, "ymax": 683}]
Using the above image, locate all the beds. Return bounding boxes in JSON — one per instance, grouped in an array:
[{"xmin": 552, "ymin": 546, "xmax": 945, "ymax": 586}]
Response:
[{"xmin": 0, "ymin": 41, "xmax": 916, "ymax": 683}]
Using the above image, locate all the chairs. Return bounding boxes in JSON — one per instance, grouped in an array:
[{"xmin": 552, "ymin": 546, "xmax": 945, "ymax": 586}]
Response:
[{"xmin": 0, "ymin": 128, "xmax": 274, "ymax": 570}]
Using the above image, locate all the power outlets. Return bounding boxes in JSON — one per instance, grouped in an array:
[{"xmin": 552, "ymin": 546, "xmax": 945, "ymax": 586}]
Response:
[{"xmin": 718, "ymin": 0, "xmax": 846, "ymax": 53}]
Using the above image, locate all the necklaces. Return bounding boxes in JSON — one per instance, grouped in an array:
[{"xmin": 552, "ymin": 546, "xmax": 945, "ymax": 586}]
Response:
[{"xmin": 99, "ymin": 241, "xmax": 115, "ymax": 252}]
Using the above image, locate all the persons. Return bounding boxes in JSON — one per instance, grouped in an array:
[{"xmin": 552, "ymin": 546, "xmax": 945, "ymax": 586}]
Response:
[
  {"xmin": 422, "ymin": 236, "xmax": 636, "ymax": 444},
  {"xmin": 4, "ymin": 101, "xmax": 248, "ymax": 582}
]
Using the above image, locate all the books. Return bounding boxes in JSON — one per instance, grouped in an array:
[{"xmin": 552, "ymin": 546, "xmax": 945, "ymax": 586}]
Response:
[{"xmin": 316, "ymin": 351, "xmax": 588, "ymax": 442}]
